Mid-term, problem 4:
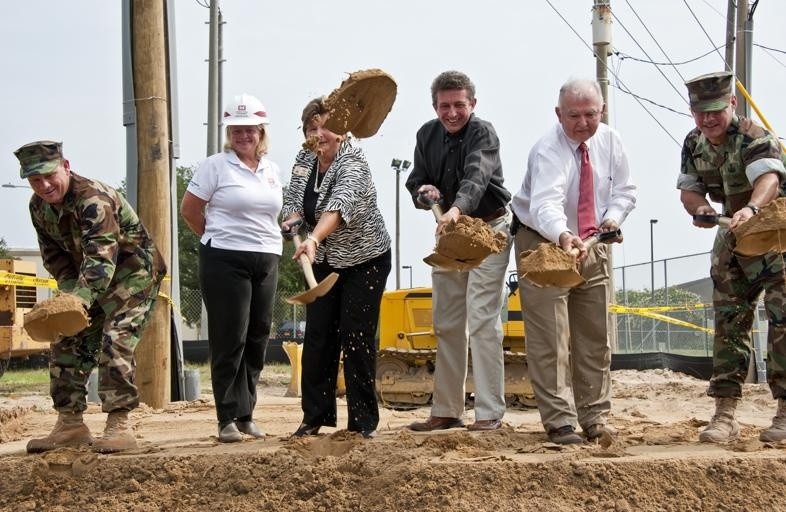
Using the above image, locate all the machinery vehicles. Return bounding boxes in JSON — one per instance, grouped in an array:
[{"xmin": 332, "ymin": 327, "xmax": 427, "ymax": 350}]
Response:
[
  {"xmin": 281, "ymin": 273, "xmax": 536, "ymax": 410},
  {"xmin": 0, "ymin": 258, "xmax": 57, "ymax": 373}
]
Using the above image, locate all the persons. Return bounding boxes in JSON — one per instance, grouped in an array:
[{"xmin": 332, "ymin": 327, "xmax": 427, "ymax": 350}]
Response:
[
  {"xmin": 676, "ymin": 71, "xmax": 786, "ymax": 445},
  {"xmin": 181, "ymin": 94, "xmax": 284, "ymax": 441},
  {"xmin": 14, "ymin": 140, "xmax": 165, "ymax": 452},
  {"xmin": 405, "ymin": 68, "xmax": 514, "ymax": 432},
  {"xmin": 281, "ymin": 94, "xmax": 392, "ymax": 438},
  {"xmin": 509, "ymin": 78, "xmax": 637, "ymax": 445}
]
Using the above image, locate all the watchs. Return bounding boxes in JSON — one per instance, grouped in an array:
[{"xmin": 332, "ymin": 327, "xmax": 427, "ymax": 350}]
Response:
[{"xmin": 744, "ymin": 205, "xmax": 760, "ymax": 215}]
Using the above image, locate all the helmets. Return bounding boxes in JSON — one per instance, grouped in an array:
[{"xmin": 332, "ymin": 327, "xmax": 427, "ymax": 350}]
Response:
[{"xmin": 223, "ymin": 96, "xmax": 267, "ymax": 125}]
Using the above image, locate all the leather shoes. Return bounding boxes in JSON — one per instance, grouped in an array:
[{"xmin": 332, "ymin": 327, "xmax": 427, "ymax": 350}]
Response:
[
  {"xmin": 411, "ymin": 415, "xmax": 464, "ymax": 432},
  {"xmin": 238, "ymin": 416, "xmax": 266, "ymax": 438},
  {"xmin": 467, "ymin": 421, "xmax": 501, "ymax": 430},
  {"xmin": 289, "ymin": 418, "xmax": 320, "ymax": 439},
  {"xmin": 219, "ymin": 418, "xmax": 243, "ymax": 443}
]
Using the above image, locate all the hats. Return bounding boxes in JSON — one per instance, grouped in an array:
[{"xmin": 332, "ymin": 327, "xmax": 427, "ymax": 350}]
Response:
[
  {"xmin": 13, "ymin": 142, "xmax": 63, "ymax": 179},
  {"xmin": 686, "ymin": 73, "xmax": 733, "ymax": 112}
]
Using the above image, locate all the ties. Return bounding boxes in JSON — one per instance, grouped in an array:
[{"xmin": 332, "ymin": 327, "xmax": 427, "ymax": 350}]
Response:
[{"xmin": 578, "ymin": 143, "xmax": 600, "ymax": 239}]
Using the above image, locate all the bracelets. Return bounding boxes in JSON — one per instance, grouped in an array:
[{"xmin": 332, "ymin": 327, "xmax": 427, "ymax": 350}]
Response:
[{"xmin": 307, "ymin": 236, "xmax": 319, "ymax": 249}]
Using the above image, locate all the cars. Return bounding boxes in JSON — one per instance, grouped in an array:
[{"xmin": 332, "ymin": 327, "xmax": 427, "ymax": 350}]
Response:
[{"xmin": 275, "ymin": 320, "xmax": 305, "ymax": 340}]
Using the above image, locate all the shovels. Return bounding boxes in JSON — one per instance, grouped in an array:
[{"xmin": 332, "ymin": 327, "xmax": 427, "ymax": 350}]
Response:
[
  {"xmin": 21, "ymin": 309, "xmax": 89, "ymax": 342},
  {"xmin": 692, "ymin": 213, "xmax": 776, "ymax": 259},
  {"xmin": 280, "ymin": 217, "xmax": 339, "ymax": 306},
  {"xmin": 419, "ymin": 189, "xmax": 481, "ymax": 273},
  {"xmin": 519, "ymin": 229, "xmax": 622, "ymax": 289}
]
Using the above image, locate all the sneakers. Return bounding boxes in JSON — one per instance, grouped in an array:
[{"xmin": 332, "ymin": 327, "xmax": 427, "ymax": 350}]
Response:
[
  {"xmin": 584, "ymin": 421, "xmax": 619, "ymax": 438},
  {"xmin": 548, "ymin": 425, "xmax": 583, "ymax": 445}
]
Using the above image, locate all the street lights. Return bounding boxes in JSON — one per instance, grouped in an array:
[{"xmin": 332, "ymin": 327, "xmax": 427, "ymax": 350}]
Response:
[
  {"xmin": 390, "ymin": 157, "xmax": 412, "ymax": 289},
  {"xmin": 1, "ymin": 183, "xmax": 30, "ymax": 191},
  {"xmin": 402, "ymin": 263, "xmax": 413, "ymax": 283},
  {"xmin": 650, "ymin": 217, "xmax": 658, "ymax": 353}
]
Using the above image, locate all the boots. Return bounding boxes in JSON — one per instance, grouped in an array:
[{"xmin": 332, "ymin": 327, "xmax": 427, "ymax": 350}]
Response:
[
  {"xmin": 92, "ymin": 412, "xmax": 136, "ymax": 452},
  {"xmin": 760, "ymin": 400, "xmax": 786, "ymax": 442},
  {"xmin": 699, "ymin": 397, "xmax": 741, "ymax": 444},
  {"xmin": 27, "ymin": 412, "xmax": 90, "ymax": 451}
]
